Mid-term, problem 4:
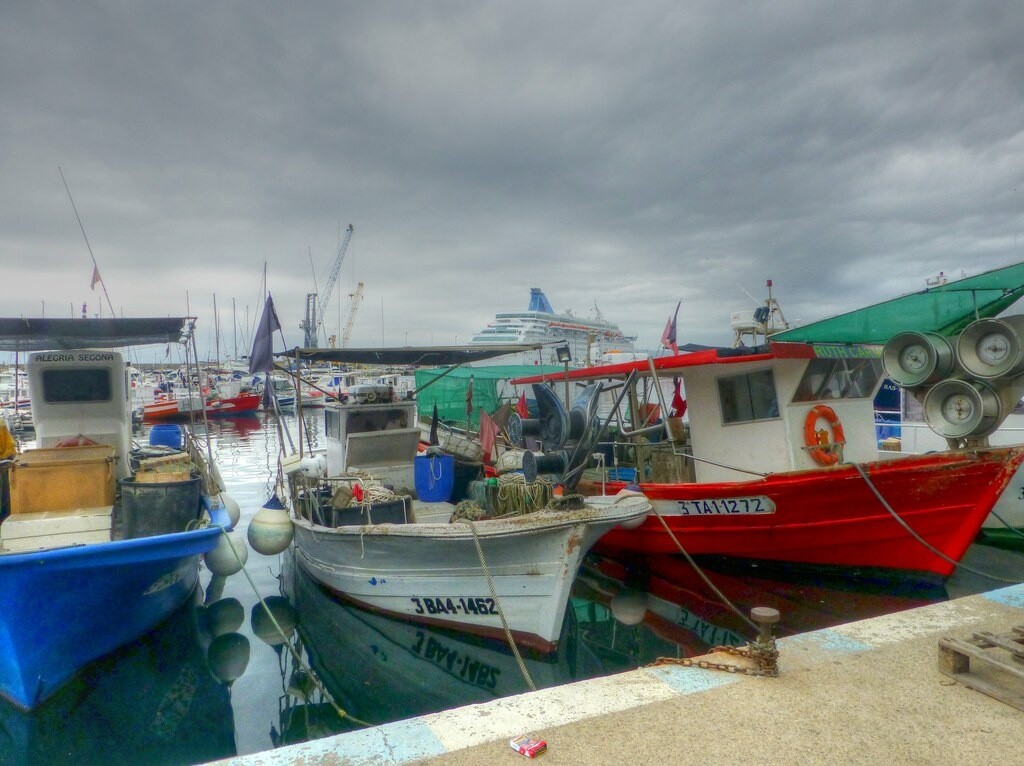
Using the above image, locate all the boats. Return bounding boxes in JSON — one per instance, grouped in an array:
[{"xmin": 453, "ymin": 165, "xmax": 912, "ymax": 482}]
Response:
[
  {"xmin": 570, "ymin": 546, "xmax": 1024, "ymax": 656},
  {"xmin": 281, "ymin": 549, "xmax": 610, "ymax": 726},
  {"xmin": 0, "ymin": 317, "xmax": 232, "ymax": 712},
  {"xmin": 415, "ymin": 264, "xmax": 1023, "ymax": 588},
  {"xmin": 467, "ymin": 288, "xmax": 638, "ymax": 369},
  {"xmin": 278, "ymin": 383, "xmax": 652, "ymax": 654}
]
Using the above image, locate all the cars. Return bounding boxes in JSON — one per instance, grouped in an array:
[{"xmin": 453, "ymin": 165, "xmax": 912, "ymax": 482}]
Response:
[{"xmin": 404, "ymin": 365, "xmax": 415, "ymax": 371}]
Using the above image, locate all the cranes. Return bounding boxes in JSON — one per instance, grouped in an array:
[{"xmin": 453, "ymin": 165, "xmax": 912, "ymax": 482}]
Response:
[
  {"xmin": 342, "ymin": 282, "xmax": 365, "ymax": 348},
  {"xmin": 299, "ymin": 224, "xmax": 355, "ymax": 348}
]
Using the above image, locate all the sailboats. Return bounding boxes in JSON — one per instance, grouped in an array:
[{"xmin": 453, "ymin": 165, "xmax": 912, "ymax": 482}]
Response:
[{"xmin": 0, "ymin": 292, "xmax": 401, "ymax": 428}]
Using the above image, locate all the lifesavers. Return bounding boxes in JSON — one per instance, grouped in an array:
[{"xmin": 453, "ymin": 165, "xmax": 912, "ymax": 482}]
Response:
[
  {"xmin": 55, "ymin": 436, "xmax": 98, "ymax": 447},
  {"xmin": 803, "ymin": 406, "xmax": 846, "ymax": 466}
]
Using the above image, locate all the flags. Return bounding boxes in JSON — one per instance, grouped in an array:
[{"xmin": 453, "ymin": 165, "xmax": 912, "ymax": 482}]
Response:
[
  {"xmin": 515, "ymin": 392, "xmax": 528, "ymax": 419},
  {"xmin": 665, "ymin": 302, "xmax": 681, "ymax": 344},
  {"xmin": 248, "ymin": 295, "xmax": 280, "ymax": 375},
  {"xmin": 89, "ymin": 267, "xmax": 100, "ymax": 292},
  {"xmin": 478, "ymin": 409, "xmax": 500, "ymax": 454},
  {"xmin": 490, "ymin": 400, "xmax": 511, "ymax": 430},
  {"xmin": 661, "ymin": 321, "xmax": 680, "ymax": 357}
]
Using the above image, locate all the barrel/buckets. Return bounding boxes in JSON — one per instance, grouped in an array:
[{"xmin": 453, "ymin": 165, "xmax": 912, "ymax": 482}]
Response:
[
  {"xmin": 120, "ymin": 475, "xmax": 201, "ymax": 539},
  {"xmin": 129, "ymin": 448, "xmax": 182, "ymax": 456},
  {"xmin": 130, "ymin": 455, "xmax": 168, "ymax": 468},
  {"xmin": 414, "ymin": 455, "xmax": 454, "ymax": 502},
  {"xmin": 132, "ymin": 468, "xmax": 202, "ymax": 476},
  {"xmin": 150, "ymin": 424, "xmax": 182, "ymax": 447},
  {"xmin": 298, "ymin": 490, "xmax": 411, "ymax": 528}
]
